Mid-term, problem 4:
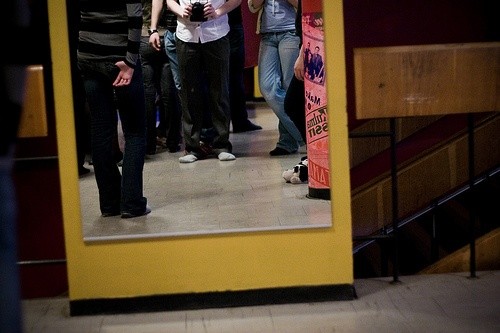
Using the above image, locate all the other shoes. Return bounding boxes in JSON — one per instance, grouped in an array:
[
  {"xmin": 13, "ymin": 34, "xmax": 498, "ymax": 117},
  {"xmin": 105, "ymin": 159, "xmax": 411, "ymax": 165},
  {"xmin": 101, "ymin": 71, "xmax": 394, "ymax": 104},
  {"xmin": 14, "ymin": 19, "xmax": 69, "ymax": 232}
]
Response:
[
  {"xmin": 179, "ymin": 154, "xmax": 197, "ymax": 163},
  {"xmin": 102, "ymin": 205, "xmax": 151, "ymax": 219},
  {"xmin": 269, "ymin": 146, "xmax": 298, "ymax": 155},
  {"xmin": 282, "ymin": 156, "xmax": 308, "ymax": 184},
  {"xmin": 233, "ymin": 119, "xmax": 262, "ymax": 133},
  {"xmin": 218, "ymin": 152, "xmax": 236, "ymax": 161},
  {"xmin": 78, "ymin": 166, "xmax": 91, "ymax": 177}
]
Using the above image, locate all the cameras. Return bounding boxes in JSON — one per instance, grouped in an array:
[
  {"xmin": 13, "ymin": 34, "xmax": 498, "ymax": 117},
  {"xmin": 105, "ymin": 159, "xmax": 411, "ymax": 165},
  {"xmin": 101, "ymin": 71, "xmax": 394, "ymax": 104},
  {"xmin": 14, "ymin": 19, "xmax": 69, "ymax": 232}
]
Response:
[{"xmin": 190, "ymin": 2, "xmax": 208, "ymax": 22}]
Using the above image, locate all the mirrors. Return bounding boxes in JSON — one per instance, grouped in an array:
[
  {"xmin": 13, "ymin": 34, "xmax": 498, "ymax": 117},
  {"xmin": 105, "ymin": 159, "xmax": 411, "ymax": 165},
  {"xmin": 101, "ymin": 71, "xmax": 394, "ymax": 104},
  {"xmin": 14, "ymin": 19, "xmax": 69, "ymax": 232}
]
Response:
[{"xmin": 66, "ymin": 1, "xmax": 332, "ymax": 242}]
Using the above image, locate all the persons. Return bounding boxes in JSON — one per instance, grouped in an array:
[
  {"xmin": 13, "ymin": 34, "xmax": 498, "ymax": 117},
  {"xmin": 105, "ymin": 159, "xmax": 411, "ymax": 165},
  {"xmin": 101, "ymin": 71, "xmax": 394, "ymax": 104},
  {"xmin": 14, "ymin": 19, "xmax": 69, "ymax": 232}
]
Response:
[
  {"xmin": 0, "ymin": 1, "xmax": 32, "ymax": 333},
  {"xmin": 67, "ymin": 0, "xmax": 181, "ymax": 218},
  {"xmin": 304, "ymin": 42, "xmax": 323, "ymax": 80},
  {"xmin": 166, "ymin": 0, "xmax": 262, "ymax": 162},
  {"xmin": 249, "ymin": 0, "xmax": 308, "ymax": 183}
]
